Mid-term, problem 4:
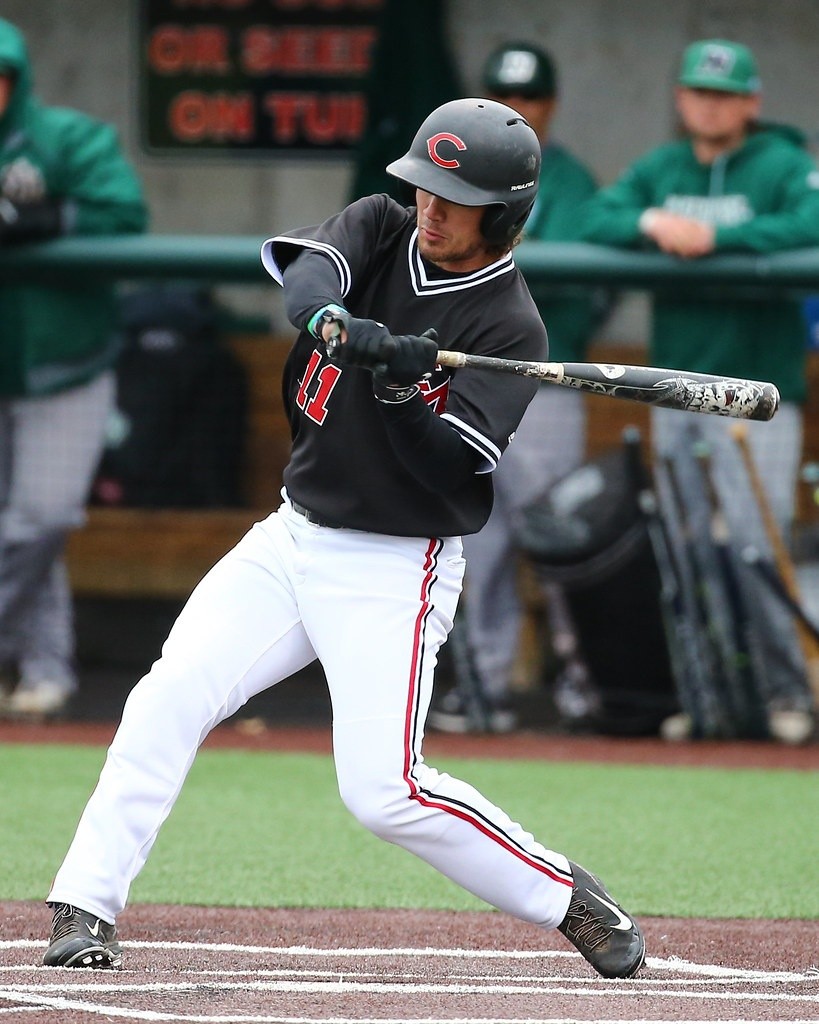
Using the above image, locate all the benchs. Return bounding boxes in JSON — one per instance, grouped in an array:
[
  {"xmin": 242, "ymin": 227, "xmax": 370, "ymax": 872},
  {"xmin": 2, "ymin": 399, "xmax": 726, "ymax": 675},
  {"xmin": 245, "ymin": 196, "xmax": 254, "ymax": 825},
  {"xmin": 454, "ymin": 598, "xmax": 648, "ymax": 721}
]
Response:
[{"xmin": 63, "ymin": 335, "xmax": 819, "ymax": 696}]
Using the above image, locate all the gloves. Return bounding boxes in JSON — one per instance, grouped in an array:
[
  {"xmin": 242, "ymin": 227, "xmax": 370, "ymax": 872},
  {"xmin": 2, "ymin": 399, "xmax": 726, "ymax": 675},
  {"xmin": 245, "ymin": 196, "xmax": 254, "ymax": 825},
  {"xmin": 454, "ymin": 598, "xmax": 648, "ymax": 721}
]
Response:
[
  {"xmin": 373, "ymin": 328, "xmax": 438, "ymax": 388},
  {"xmin": 318, "ymin": 314, "xmax": 396, "ymax": 380},
  {"xmin": 0, "ymin": 199, "xmax": 64, "ymax": 243}
]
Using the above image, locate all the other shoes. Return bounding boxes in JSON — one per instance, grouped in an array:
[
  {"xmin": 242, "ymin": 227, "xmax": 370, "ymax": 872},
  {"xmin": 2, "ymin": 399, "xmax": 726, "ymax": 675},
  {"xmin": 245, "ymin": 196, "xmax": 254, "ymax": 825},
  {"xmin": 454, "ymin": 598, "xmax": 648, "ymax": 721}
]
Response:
[{"xmin": 9, "ymin": 683, "xmax": 70, "ymax": 720}]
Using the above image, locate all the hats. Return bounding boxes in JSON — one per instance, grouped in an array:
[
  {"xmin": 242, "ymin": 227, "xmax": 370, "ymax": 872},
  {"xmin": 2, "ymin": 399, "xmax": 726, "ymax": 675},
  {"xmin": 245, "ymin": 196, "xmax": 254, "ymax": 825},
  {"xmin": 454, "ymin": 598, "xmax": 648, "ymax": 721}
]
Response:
[
  {"xmin": 679, "ymin": 39, "xmax": 759, "ymax": 93},
  {"xmin": 482, "ymin": 43, "xmax": 554, "ymax": 91}
]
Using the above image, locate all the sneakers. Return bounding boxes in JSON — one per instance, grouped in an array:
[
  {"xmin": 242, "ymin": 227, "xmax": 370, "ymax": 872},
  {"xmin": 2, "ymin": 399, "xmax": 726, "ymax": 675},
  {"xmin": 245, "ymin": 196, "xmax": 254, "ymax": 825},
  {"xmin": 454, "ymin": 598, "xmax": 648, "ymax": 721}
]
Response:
[
  {"xmin": 557, "ymin": 860, "xmax": 645, "ymax": 979},
  {"xmin": 43, "ymin": 902, "xmax": 121, "ymax": 969}
]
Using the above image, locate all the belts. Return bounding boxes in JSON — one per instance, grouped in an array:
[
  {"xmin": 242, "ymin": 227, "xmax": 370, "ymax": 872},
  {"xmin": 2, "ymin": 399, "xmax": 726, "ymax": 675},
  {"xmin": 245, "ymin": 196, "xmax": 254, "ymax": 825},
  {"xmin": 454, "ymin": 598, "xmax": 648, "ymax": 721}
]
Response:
[{"xmin": 289, "ymin": 498, "xmax": 351, "ymax": 528}]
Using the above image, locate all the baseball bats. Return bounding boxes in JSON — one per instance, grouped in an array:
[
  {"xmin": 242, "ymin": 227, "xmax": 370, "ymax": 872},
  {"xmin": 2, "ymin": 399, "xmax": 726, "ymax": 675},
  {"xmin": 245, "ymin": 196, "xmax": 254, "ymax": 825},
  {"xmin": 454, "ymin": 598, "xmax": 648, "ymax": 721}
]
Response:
[
  {"xmin": 326, "ymin": 335, "xmax": 781, "ymax": 423},
  {"xmin": 493, "ymin": 416, "xmax": 819, "ymax": 741}
]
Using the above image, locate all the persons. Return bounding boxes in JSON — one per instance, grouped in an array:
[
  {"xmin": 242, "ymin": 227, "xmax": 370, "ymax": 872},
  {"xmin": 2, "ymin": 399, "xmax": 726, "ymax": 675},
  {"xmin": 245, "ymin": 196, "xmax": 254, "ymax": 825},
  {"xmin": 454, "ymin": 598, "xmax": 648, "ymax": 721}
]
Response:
[
  {"xmin": 427, "ymin": 33, "xmax": 817, "ymax": 742},
  {"xmin": 43, "ymin": 99, "xmax": 649, "ymax": 983},
  {"xmin": 0, "ymin": 18, "xmax": 149, "ymax": 718}
]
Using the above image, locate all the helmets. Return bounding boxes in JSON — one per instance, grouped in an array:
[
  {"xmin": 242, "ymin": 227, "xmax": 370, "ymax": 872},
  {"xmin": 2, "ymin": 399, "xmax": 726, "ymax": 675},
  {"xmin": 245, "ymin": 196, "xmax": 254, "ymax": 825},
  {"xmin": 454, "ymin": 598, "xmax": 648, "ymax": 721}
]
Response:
[{"xmin": 386, "ymin": 98, "xmax": 541, "ymax": 242}]
